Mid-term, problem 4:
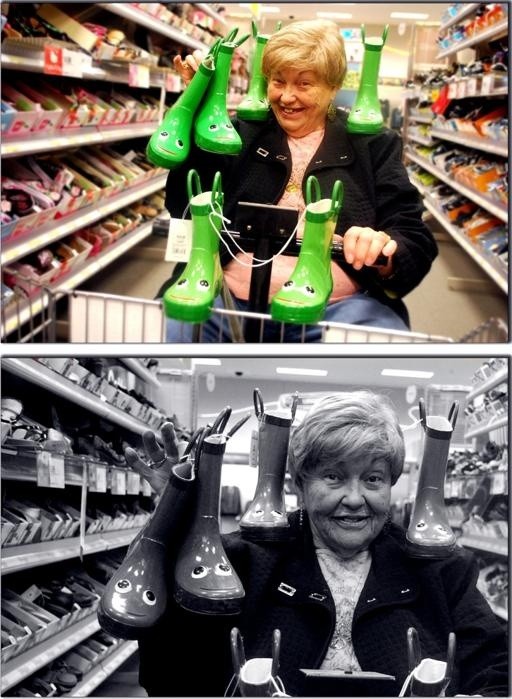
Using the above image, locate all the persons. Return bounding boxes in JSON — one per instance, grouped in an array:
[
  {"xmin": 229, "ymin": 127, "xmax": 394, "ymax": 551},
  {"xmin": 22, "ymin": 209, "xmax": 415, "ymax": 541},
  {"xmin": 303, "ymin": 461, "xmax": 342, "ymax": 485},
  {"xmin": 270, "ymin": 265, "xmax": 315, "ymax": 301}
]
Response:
[
  {"xmin": 148, "ymin": 19, "xmax": 440, "ymax": 343},
  {"xmin": 123, "ymin": 390, "xmax": 510, "ymax": 697}
]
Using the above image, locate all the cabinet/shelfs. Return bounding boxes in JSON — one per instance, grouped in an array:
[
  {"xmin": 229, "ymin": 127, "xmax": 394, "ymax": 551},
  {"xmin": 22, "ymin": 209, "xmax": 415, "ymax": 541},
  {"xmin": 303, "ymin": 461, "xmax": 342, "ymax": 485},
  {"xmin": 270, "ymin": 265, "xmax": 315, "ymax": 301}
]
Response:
[
  {"xmin": 3, "ymin": 0, "xmax": 249, "ymax": 337},
  {"xmin": 3, "ymin": 358, "xmax": 171, "ymax": 698},
  {"xmin": 439, "ymin": 365, "xmax": 508, "ymax": 558},
  {"xmin": 401, "ymin": 0, "xmax": 509, "ymax": 296}
]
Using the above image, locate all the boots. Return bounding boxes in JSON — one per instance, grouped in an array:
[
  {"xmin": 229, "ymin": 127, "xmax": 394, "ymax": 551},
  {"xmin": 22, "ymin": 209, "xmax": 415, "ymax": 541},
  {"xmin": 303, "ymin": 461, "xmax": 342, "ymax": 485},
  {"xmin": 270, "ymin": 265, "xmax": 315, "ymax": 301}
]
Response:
[
  {"xmin": 146, "ymin": 19, "xmax": 282, "ymax": 170},
  {"xmin": 346, "ymin": 23, "xmax": 388, "ymax": 136},
  {"xmin": 408, "ymin": 400, "xmax": 457, "ymax": 562},
  {"xmin": 164, "ymin": 171, "xmax": 223, "ymax": 325},
  {"xmin": 273, "ymin": 175, "xmax": 344, "ymax": 324},
  {"xmin": 98, "ymin": 388, "xmax": 301, "ymax": 634}
]
